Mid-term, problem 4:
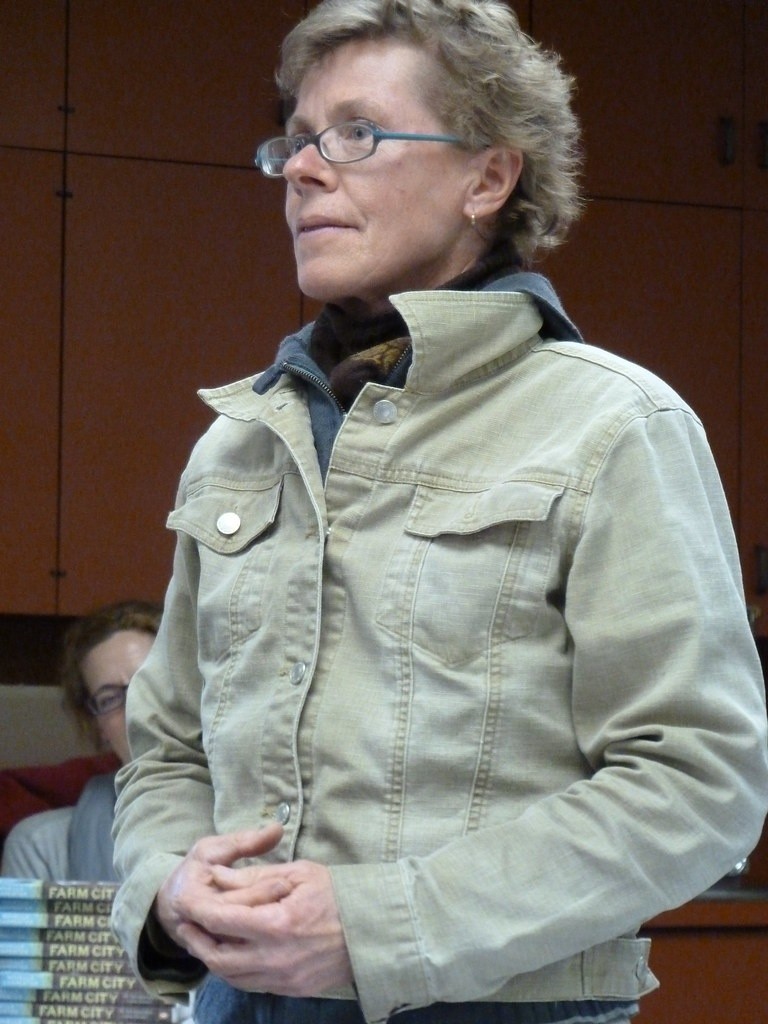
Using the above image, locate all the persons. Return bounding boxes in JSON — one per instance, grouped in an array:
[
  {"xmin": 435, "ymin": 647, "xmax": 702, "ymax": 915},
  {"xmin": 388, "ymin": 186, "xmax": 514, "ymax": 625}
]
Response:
[
  {"xmin": 0, "ymin": 601, "xmax": 162, "ymax": 881},
  {"xmin": 111, "ymin": 0, "xmax": 768, "ymax": 1024}
]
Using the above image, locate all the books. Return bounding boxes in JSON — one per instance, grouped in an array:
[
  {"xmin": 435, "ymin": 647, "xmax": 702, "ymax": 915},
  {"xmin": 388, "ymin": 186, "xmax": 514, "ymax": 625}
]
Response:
[{"xmin": 0, "ymin": 878, "xmax": 173, "ymax": 1024}]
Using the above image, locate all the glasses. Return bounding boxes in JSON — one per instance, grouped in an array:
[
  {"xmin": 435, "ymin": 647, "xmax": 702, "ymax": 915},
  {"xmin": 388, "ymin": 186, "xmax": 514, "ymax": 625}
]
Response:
[
  {"xmin": 84, "ymin": 686, "xmax": 126, "ymax": 716},
  {"xmin": 254, "ymin": 122, "xmax": 465, "ymax": 177}
]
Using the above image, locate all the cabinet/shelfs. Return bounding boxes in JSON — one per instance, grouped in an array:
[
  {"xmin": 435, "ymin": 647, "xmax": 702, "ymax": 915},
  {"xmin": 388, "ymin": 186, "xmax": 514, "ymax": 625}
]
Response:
[{"xmin": 1, "ymin": 0, "xmax": 768, "ymax": 643}]
